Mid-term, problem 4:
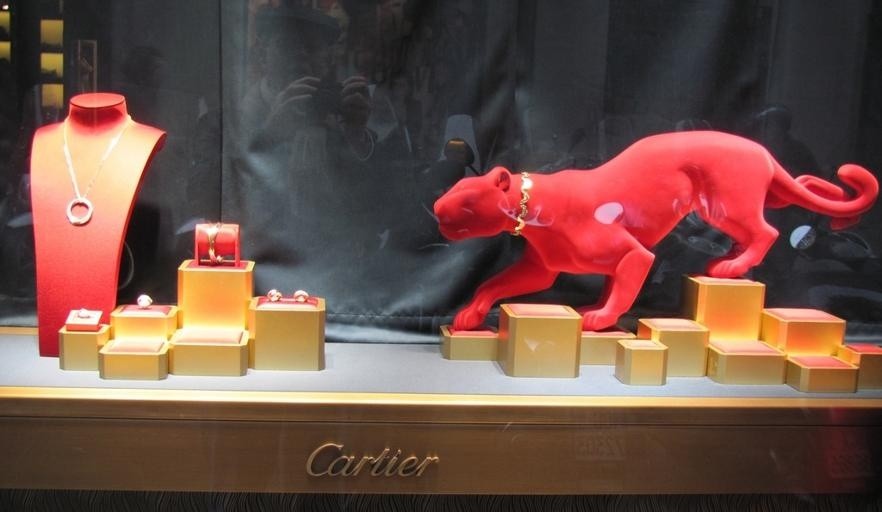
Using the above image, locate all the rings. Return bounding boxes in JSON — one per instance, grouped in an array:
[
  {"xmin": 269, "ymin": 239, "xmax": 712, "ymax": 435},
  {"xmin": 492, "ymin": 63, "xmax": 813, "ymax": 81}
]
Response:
[
  {"xmin": 293, "ymin": 289, "xmax": 309, "ymax": 303},
  {"xmin": 137, "ymin": 294, "xmax": 154, "ymax": 308},
  {"xmin": 266, "ymin": 289, "xmax": 282, "ymax": 301},
  {"xmin": 77, "ymin": 308, "xmax": 91, "ymax": 319}
]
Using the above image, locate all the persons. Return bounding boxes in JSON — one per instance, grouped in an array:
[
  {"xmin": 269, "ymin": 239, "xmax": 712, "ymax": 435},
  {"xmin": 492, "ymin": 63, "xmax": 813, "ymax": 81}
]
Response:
[{"xmin": 222, "ymin": 3, "xmax": 412, "ymax": 327}]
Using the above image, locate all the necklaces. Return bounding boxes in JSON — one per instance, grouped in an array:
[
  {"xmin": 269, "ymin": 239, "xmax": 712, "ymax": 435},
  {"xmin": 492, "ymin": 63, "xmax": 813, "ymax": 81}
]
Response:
[
  {"xmin": 61, "ymin": 117, "xmax": 134, "ymax": 227},
  {"xmin": 503, "ymin": 169, "xmax": 534, "ymax": 239}
]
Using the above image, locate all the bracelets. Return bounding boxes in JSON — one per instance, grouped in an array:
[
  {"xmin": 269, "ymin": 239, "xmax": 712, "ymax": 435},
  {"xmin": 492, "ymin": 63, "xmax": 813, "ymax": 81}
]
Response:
[{"xmin": 208, "ymin": 226, "xmax": 223, "ymax": 264}]
